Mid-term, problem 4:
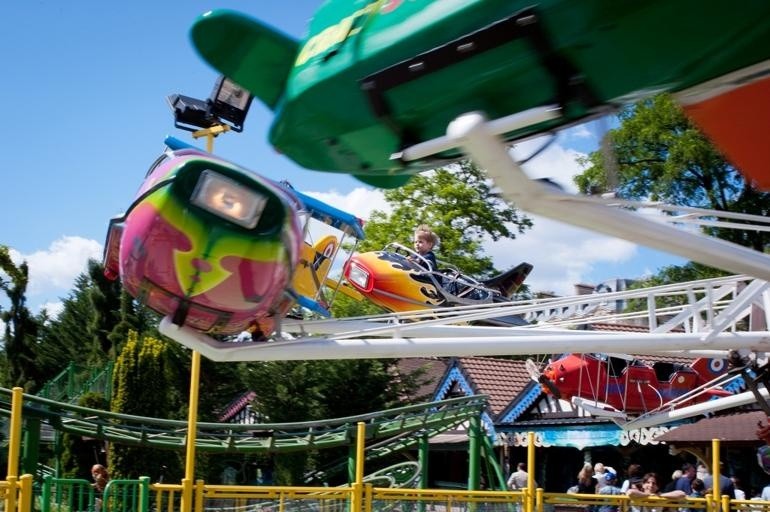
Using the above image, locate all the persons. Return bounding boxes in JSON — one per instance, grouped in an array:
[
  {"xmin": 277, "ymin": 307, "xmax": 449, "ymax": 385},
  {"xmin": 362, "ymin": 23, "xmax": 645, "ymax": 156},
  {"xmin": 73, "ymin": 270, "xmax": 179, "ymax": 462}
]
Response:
[
  {"xmin": 506, "ymin": 462, "xmax": 537, "ymax": 491},
  {"xmin": 89, "ymin": 464, "xmax": 111, "ymax": 495},
  {"xmin": 577, "ymin": 461, "xmax": 769, "ymax": 501},
  {"xmin": 414, "ymin": 230, "xmax": 440, "ymax": 282}
]
